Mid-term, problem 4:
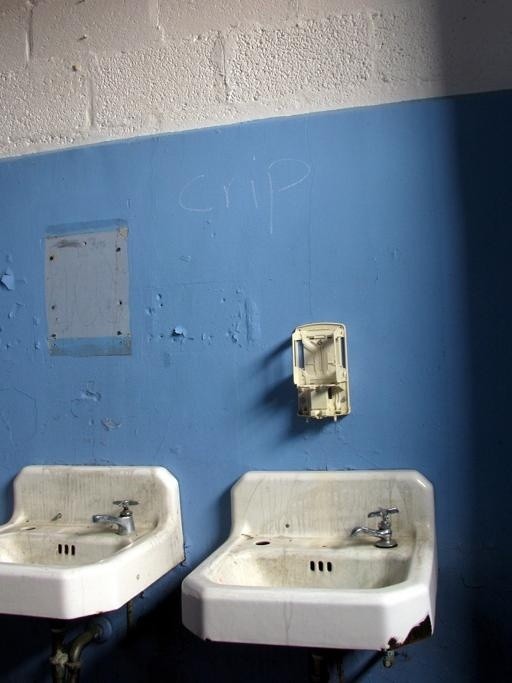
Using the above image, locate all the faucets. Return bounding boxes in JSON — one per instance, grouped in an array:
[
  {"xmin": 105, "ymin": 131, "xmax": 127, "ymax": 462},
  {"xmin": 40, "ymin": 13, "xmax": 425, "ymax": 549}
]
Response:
[
  {"xmin": 349, "ymin": 505, "xmax": 399, "ymax": 547},
  {"xmin": 92, "ymin": 500, "xmax": 140, "ymax": 535}
]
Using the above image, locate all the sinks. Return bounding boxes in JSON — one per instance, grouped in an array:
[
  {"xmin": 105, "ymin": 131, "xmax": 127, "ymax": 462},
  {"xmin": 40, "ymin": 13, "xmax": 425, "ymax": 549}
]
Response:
[
  {"xmin": 180, "ymin": 471, "xmax": 438, "ymax": 651},
  {"xmin": 1, "ymin": 464, "xmax": 185, "ymax": 620}
]
